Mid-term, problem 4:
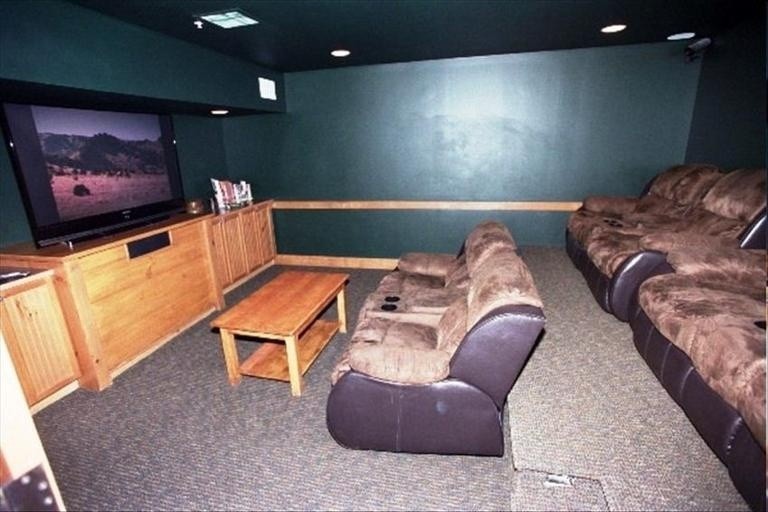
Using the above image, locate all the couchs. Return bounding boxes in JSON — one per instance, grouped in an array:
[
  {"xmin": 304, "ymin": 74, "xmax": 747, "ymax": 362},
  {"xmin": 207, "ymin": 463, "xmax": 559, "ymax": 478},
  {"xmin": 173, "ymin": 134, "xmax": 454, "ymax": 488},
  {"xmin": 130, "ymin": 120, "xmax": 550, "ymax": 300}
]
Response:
[
  {"xmin": 568, "ymin": 163, "xmax": 767, "ymax": 324},
  {"xmin": 626, "ymin": 246, "xmax": 766, "ymax": 511},
  {"xmin": 328, "ymin": 217, "xmax": 546, "ymax": 458}
]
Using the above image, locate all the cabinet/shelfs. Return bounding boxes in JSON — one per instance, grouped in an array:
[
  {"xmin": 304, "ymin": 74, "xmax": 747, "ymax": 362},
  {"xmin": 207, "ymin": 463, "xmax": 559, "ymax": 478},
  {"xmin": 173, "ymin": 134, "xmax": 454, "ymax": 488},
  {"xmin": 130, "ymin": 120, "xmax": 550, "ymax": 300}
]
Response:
[{"xmin": 1, "ymin": 198, "xmax": 281, "ymax": 416}]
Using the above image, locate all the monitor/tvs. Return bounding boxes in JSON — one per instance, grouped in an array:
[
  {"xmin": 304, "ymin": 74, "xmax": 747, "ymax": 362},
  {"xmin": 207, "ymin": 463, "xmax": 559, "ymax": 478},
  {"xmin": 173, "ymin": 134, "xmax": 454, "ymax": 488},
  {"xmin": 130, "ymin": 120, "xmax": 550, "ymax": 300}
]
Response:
[{"xmin": 1, "ymin": 88, "xmax": 188, "ymax": 249}]
